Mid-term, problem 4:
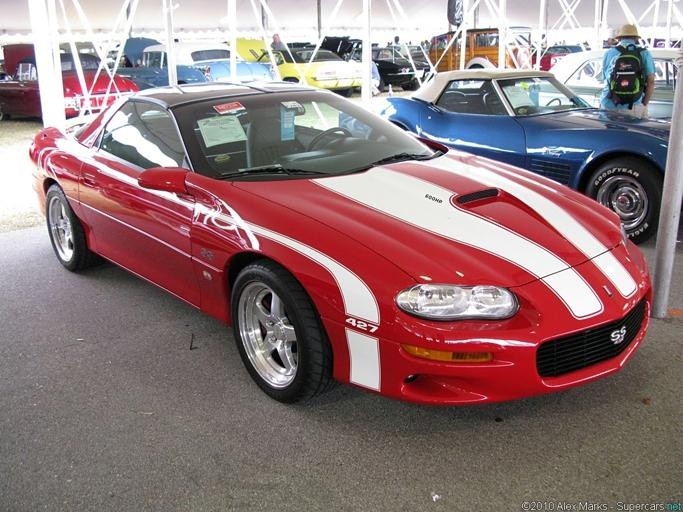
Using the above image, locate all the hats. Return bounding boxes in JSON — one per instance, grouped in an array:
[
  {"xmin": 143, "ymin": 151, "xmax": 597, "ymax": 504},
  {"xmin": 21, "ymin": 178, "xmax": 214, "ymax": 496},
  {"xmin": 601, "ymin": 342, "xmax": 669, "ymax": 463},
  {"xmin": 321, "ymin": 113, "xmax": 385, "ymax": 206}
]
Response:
[{"xmin": 615, "ymin": 24, "xmax": 641, "ymax": 39}]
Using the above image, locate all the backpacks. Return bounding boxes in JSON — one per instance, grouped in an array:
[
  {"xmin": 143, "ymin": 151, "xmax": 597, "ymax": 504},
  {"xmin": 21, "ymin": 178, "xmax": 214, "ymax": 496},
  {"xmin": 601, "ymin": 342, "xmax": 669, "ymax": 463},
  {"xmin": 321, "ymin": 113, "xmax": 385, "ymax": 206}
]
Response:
[{"xmin": 609, "ymin": 45, "xmax": 647, "ymax": 105}]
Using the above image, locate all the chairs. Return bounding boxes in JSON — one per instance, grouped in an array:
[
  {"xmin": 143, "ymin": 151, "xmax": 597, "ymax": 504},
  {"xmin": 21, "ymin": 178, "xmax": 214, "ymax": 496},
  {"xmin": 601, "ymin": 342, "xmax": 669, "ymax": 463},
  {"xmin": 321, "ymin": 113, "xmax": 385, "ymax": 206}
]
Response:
[
  {"xmin": 246, "ymin": 114, "xmax": 303, "ymax": 167},
  {"xmin": 481, "ymin": 91, "xmax": 508, "ymax": 115},
  {"xmin": 437, "ymin": 91, "xmax": 470, "ymax": 113}
]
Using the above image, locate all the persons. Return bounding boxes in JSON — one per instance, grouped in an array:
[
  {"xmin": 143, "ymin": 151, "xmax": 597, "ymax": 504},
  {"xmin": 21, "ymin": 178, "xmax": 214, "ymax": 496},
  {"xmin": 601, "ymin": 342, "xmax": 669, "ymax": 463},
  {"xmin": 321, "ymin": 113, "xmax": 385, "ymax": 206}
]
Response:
[
  {"xmin": 270, "ymin": 35, "xmax": 285, "ymax": 51},
  {"xmin": 371, "ymin": 36, "xmax": 430, "ymax": 97},
  {"xmin": 597, "ymin": 23, "xmax": 654, "ymax": 123}
]
